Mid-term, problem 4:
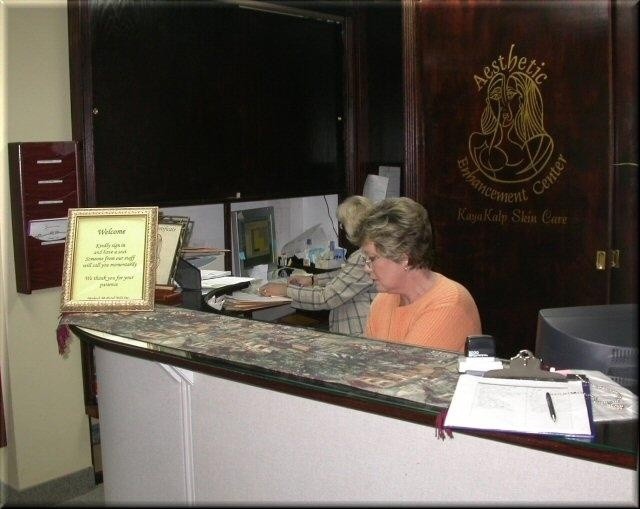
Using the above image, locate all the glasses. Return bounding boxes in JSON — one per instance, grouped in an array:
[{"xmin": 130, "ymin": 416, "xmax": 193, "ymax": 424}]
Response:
[{"xmin": 360, "ymin": 254, "xmax": 385, "ymax": 266}]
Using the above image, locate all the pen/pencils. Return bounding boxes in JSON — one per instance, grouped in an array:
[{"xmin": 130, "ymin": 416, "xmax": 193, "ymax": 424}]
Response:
[{"xmin": 546, "ymin": 393, "xmax": 556, "ymax": 422}]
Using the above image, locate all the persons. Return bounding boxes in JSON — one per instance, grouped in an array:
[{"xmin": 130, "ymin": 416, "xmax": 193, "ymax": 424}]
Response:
[
  {"xmin": 258, "ymin": 194, "xmax": 386, "ymax": 339},
  {"xmin": 349, "ymin": 194, "xmax": 483, "ymax": 358}
]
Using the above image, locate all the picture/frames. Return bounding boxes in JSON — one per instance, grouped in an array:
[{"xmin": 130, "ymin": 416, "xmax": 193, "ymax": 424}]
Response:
[{"xmin": 60, "ymin": 207, "xmax": 158, "ymax": 313}]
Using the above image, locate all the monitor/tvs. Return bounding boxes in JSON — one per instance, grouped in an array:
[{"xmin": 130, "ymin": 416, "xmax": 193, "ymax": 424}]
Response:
[
  {"xmin": 230, "ymin": 205, "xmax": 278, "ymax": 276},
  {"xmin": 533, "ymin": 302, "xmax": 640, "ymax": 394}
]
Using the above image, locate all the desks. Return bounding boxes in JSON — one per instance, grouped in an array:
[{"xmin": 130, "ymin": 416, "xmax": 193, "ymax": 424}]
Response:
[{"xmin": 200, "ymin": 276, "xmax": 293, "ymax": 318}]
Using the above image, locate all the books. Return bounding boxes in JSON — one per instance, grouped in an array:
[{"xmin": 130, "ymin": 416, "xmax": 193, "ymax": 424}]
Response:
[{"xmin": 196, "ymin": 263, "xmax": 295, "ymax": 315}]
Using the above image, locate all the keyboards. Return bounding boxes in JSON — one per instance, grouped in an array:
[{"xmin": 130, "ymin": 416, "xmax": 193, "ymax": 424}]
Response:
[{"xmin": 241, "ymin": 277, "xmax": 292, "ymax": 297}]
